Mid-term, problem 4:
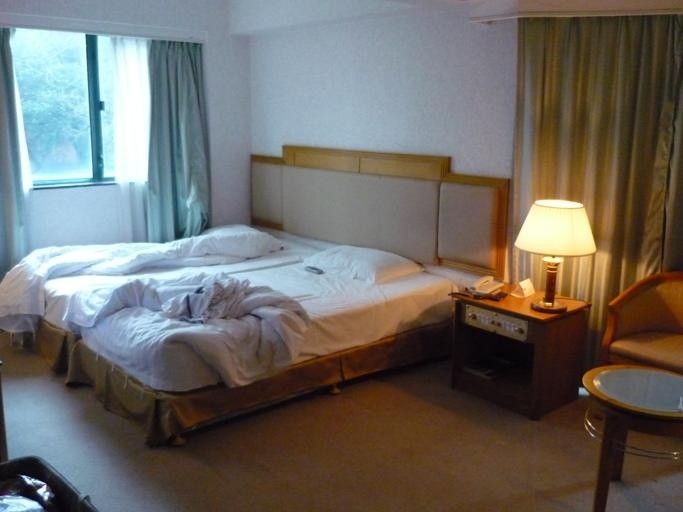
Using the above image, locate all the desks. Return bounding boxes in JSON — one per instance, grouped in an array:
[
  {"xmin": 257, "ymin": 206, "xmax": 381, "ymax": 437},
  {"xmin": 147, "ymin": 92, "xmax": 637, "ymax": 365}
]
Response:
[{"xmin": 581, "ymin": 364, "xmax": 683, "ymax": 511}]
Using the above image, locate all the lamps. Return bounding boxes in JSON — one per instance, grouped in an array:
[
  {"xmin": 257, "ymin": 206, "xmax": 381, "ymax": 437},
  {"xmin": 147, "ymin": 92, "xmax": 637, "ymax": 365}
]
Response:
[{"xmin": 513, "ymin": 200, "xmax": 598, "ymax": 308}]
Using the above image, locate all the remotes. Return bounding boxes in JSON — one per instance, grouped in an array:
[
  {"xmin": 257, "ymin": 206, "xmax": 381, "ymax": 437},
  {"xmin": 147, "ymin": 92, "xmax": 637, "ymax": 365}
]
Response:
[{"xmin": 304, "ymin": 265, "xmax": 324, "ymax": 275}]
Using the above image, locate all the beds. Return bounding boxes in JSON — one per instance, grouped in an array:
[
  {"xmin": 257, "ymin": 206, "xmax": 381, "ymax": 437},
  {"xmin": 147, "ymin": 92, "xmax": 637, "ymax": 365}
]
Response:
[{"xmin": 0, "ymin": 145, "xmax": 508, "ymax": 455}]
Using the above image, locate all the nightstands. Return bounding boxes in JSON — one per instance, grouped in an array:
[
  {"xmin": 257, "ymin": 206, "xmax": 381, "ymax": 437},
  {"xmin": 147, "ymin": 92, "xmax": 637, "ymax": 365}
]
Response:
[{"xmin": 447, "ymin": 285, "xmax": 591, "ymax": 419}]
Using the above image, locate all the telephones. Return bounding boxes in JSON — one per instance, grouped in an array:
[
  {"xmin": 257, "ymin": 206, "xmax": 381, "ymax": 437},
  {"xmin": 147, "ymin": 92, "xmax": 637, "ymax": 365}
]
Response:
[{"xmin": 466, "ymin": 275, "xmax": 505, "ymax": 299}]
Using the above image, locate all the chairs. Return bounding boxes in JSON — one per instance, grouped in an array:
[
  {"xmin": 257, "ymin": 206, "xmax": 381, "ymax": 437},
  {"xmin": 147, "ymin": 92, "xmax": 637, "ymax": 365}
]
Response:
[{"xmin": 598, "ymin": 271, "xmax": 683, "ymax": 383}]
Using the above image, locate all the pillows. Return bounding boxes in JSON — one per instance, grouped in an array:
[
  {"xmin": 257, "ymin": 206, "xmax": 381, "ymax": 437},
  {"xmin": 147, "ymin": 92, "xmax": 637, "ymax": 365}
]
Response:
[
  {"xmin": 201, "ymin": 224, "xmax": 282, "ymax": 255},
  {"xmin": 305, "ymin": 245, "xmax": 427, "ymax": 284}
]
